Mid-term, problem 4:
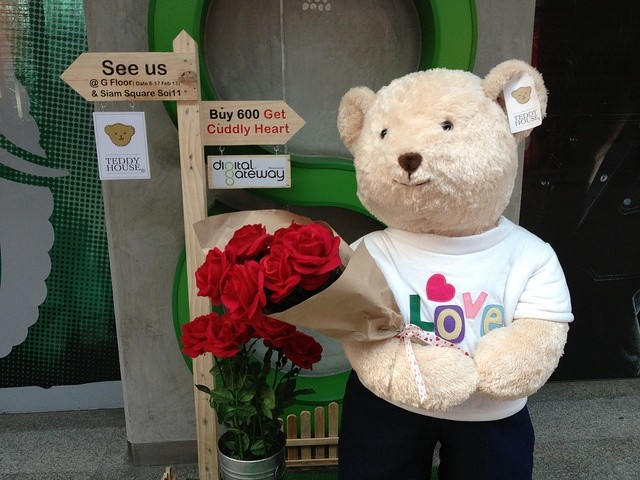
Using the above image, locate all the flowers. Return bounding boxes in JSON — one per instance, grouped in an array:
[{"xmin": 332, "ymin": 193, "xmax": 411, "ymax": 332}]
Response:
[
  {"xmin": 181, "ymin": 312, "xmax": 324, "ymax": 460},
  {"xmin": 180, "ymin": 218, "xmax": 343, "ymax": 319}
]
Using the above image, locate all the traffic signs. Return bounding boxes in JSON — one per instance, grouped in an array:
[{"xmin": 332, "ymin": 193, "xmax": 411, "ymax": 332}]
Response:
[
  {"xmin": 59, "ymin": 52, "xmax": 199, "ymax": 102},
  {"xmin": 201, "ymin": 101, "xmax": 305, "ymax": 144}
]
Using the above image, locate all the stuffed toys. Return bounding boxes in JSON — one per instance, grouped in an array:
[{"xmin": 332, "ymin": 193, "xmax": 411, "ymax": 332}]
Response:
[{"xmin": 337, "ymin": 59, "xmax": 576, "ymax": 477}]
